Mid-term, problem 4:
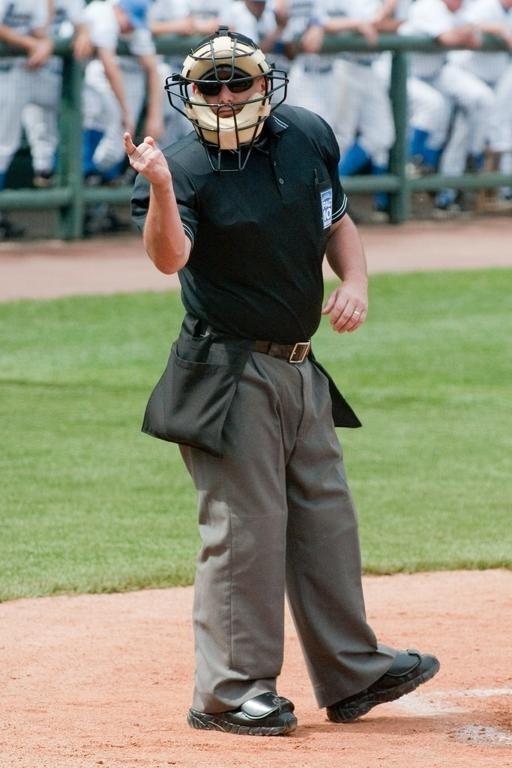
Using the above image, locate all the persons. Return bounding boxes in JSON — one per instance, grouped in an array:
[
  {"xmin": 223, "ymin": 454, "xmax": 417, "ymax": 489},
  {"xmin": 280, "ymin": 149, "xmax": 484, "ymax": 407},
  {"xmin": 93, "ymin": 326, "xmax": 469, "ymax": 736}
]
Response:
[
  {"xmin": 1, "ymin": 0, "xmax": 512, "ymax": 239},
  {"xmin": 121, "ymin": 26, "xmax": 442, "ymax": 738}
]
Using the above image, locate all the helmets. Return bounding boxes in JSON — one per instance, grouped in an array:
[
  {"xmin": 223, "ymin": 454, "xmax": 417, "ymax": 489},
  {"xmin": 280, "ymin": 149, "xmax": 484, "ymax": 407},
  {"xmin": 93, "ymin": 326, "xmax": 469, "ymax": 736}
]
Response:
[{"xmin": 164, "ymin": 27, "xmax": 289, "ymax": 174}]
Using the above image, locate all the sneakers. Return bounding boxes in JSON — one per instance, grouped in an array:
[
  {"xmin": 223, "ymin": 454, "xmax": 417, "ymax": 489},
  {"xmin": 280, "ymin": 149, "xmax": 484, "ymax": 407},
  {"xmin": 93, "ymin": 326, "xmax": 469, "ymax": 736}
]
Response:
[
  {"xmin": 185, "ymin": 690, "xmax": 298, "ymax": 736},
  {"xmin": 326, "ymin": 652, "xmax": 439, "ymax": 724}
]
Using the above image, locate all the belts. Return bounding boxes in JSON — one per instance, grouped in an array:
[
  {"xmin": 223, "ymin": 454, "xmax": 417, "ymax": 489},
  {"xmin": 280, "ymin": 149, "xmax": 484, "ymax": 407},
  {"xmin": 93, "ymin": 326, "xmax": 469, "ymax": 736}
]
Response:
[{"xmin": 252, "ymin": 339, "xmax": 316, "ymax": 364}]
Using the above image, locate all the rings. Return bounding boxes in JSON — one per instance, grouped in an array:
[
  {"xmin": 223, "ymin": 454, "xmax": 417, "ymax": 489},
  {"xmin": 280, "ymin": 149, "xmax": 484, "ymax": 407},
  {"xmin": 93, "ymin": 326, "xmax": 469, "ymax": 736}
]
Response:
[{"xmin": 354, "ymin": 311, "xmax": 360, "ymax": 315}]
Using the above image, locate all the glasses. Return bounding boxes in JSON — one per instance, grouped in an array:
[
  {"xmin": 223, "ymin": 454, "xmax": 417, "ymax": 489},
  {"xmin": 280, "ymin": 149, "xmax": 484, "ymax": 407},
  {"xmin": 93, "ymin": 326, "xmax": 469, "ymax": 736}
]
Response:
[{"xmin": 195, "ymin": 76, "xmax": 257, "ymax": 94}]
